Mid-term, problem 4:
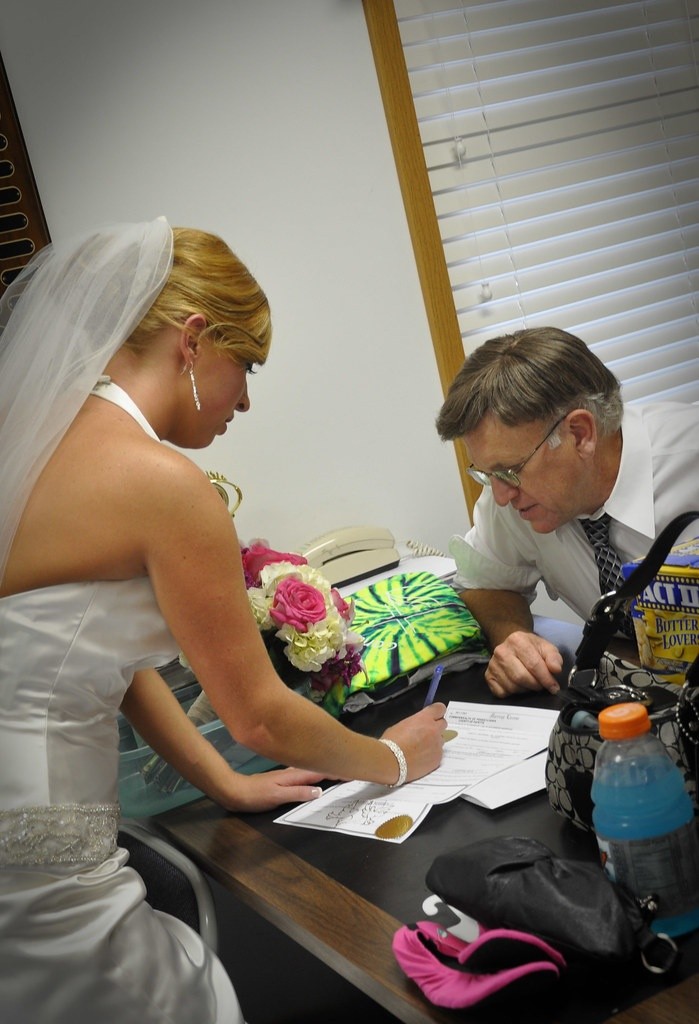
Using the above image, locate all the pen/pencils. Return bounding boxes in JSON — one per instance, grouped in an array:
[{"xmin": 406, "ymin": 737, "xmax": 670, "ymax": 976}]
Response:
[{"xmin": 423, "ymin": 664, "xmax": 445, "ymax": 708}]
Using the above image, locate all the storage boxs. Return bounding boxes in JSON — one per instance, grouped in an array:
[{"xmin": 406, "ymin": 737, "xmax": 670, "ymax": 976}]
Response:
[
  {"xmin": 621, "ymin": 534, "xmax": 699, "ymax": 686},
  {"xmin": 118, "ymin": 681, "xmax": 313, "ymax": 820}
]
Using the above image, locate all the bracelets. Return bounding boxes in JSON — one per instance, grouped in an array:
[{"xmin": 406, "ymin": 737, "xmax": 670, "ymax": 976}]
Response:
[{"xmin": 378, "ymin": 738, "xmax": 407, "ymax": 789}]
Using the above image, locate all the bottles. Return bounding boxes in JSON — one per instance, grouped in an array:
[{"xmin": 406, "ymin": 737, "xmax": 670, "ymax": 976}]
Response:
[{"xmin": 591, "ymin": 702, "xmax": 699, "ymax": 936}]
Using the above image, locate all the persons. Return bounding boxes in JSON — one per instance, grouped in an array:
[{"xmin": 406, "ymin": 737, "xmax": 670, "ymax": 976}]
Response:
[
  {"xmin": 0, "ymin": 216, "xmax": 447, "ymax": 1024},
  {"xmin": 437, "ymin": 326, "xmax": 699, "ymax": 698}
]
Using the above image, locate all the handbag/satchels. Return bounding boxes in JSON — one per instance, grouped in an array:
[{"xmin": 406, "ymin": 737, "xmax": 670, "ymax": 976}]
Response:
[
  {"xmin": 425, "ymin": 835, "xmax": 679, "ymax": 975},
  {"xmin": 546, "ymin": 513, "xmax": 699, "ymax": 861}
]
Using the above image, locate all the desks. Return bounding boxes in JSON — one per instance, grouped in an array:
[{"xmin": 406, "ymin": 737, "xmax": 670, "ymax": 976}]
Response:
[{"xmin": 147, "ymin": 683, "xmax": 699, "ymax": 1024}]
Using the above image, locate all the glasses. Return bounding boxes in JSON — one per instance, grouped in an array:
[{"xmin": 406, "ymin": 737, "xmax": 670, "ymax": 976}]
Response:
[{"xmin": 465, "ymin": 407, "xmax": 579, "ymax": 487}]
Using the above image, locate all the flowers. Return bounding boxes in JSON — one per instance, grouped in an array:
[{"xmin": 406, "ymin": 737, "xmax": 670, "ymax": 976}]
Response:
[{"xmin": 140, "ymin": 543, "xmax": 367, "ymax": 795}]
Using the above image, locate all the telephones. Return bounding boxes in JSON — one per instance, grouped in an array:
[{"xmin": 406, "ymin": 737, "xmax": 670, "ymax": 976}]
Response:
[{"xmin": 297, "ymin": 525, "xmax": 401, "ymax": 588}]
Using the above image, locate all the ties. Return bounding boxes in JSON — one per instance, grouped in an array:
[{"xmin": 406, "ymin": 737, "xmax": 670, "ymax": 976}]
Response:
[{"xmin": 578, "ymin": 514, "xmax": 634, "ymax": 637}]
